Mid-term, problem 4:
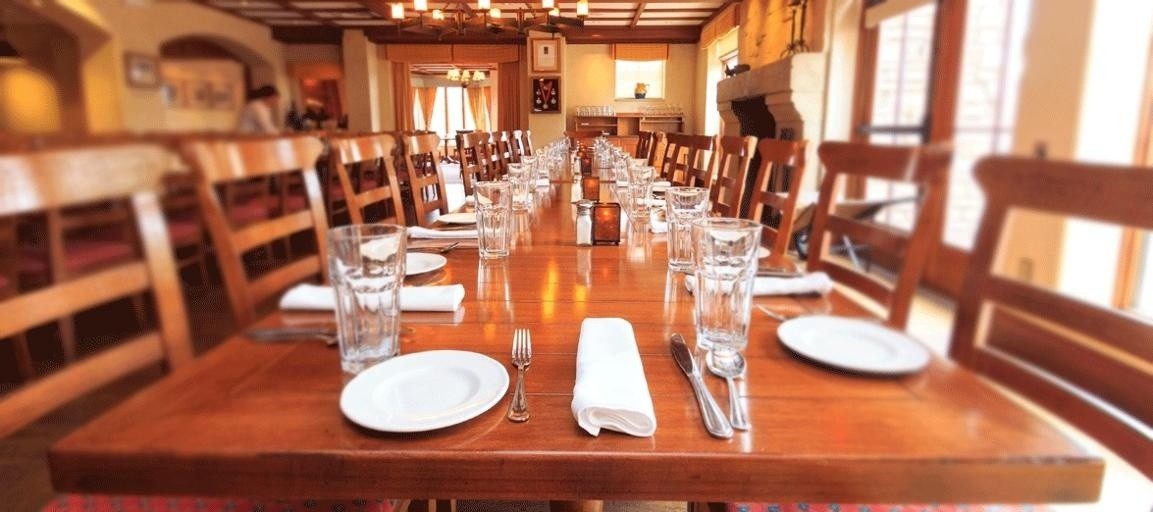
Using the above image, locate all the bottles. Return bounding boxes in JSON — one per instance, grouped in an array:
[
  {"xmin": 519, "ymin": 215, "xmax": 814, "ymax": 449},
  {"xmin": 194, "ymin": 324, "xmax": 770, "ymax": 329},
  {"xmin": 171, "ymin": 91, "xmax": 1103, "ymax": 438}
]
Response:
[
  {"xmin": 572, "ymin": 199, "xmax": 595, "ymax": 248},
  {"xmin": 569, "ymin": 148, "xmax": 582, "ymax": 200},
  {"xmin": 573, "ymin": 247, "xmax": 592, "ymax": 288}
]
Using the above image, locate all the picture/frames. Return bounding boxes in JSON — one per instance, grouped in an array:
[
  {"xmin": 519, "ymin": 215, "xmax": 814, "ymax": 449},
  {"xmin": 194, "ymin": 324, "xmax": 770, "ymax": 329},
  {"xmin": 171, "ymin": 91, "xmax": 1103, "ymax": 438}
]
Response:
[
  {"xmin": 527, "ymin": 36, "xmax": 563, "ymax": 75},
  {"xmin": 528, "ymin": 75, "xmax": 561, "ymax": 114}
]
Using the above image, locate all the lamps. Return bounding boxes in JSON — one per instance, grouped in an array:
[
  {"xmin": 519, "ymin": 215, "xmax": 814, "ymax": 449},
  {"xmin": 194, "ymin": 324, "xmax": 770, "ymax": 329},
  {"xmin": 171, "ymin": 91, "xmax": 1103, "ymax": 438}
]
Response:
[
  {"xmin": 441, "ymin": 62, "xmax": 485, "ymax": 86},
  {"xmin": 384, "ymin": 0, "xmax": 590, "ymax": 39}
]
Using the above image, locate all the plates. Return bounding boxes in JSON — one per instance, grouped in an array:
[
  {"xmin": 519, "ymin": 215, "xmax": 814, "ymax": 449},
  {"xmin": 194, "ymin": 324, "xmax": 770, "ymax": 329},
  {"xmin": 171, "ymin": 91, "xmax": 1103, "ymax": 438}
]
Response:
[
  {"xmin": 755, "ymin": 244, "xmax": 769, "ymax": 260},
  {"xmin": 337, "ymin": 348, "xmax": 510, "ymax": 435},
  {"xmin": 773, "ymin": 313, "xmax": 933, "ymax": 379},
  {"xmin": 437, "ymin": 211, "xmax": 475, "ymax": 225},
  {"xmin": 404, "ymin": 252, "xmax": 446, "ymax": 276}
]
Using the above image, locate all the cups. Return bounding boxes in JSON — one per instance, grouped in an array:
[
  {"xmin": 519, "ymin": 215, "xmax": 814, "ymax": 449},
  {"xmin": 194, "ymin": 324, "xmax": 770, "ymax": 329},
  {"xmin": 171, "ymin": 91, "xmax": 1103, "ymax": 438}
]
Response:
[
  {"xmin": 642, "ymin": 103, "xmax": 683, "ymax": 115},
  {"xmin": 324, "ymin": 223, "xmax": 405, "ymax": 374},
  {"xmin": 574, "ymin": 105, "xmax": 616, "ymax": 117},
  {"xmin": 476, "ymin": 256, "xmax": 511, "ymax": 323},
  {"xmin": 691, "ymin": 215, "xmax": 761, "ymax": 352},
  {"xmin": 473, "ymin": 138, "xmax": 565, "ymax": 260},
  {"xmin": 661, "ymin": 266, "xmax": 694, "ymax": 342},
  {"xmin": 594, "ymin": 135, "xmax": 708, "ymax": 272}
]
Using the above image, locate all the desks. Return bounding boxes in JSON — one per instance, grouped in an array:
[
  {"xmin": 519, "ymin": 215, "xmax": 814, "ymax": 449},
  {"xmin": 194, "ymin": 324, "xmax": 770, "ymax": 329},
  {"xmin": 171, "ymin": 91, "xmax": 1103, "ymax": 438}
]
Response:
[{"xmin": 45, "ymin": 150, "xmax": 1104, "ymax": 505}]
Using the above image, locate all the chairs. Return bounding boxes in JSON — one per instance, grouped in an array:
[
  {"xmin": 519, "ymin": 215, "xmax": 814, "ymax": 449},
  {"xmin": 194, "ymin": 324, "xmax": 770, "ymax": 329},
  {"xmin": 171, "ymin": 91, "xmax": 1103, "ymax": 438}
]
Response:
[{"xmin": 1, "ymin": 108, "xmax": 1152, "ymax": 508}]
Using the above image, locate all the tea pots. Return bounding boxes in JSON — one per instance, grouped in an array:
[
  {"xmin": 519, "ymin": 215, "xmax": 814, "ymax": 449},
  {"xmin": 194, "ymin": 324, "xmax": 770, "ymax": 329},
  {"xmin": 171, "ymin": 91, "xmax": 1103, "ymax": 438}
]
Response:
[{"xmin": 634, "ymin": 82, "xmax": 650, "ymax": 99}]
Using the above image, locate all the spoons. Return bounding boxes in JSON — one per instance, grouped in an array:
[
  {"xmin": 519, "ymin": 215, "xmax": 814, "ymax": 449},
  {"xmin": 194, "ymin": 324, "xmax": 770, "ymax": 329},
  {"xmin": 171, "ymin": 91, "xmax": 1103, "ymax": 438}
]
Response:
[{"xmin": 707, "ymin": 344, "xmax": 752, "ymax": 433}]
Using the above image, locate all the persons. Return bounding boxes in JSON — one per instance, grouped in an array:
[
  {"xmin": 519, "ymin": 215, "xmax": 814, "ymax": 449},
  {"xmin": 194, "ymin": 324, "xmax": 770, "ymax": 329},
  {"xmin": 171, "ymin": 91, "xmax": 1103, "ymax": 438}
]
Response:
[
  {"xmin": 238, "ymin": 84, "xmax": 296, "ymax": 141},
  {"xmin": 282, "ymin": 97, "xmax": 302, "ymax": 132},
  {"xmin": 297, "ymin": 98, "xmax": 341, "ymax": 131}
]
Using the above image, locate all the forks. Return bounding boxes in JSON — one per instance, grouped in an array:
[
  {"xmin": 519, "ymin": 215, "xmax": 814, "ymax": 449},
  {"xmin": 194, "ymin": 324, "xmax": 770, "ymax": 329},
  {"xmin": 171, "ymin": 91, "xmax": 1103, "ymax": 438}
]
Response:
[
  {"xmin": 755, "ymin": 302, "xmax": 784, "ymax": 322},
  {"xmin": 507, "ymin": 327, "xmax": 532, "ymax": 424},
  {"xmin": 411, "ymin": 240, "xmax": 462, "ymax": 252}
]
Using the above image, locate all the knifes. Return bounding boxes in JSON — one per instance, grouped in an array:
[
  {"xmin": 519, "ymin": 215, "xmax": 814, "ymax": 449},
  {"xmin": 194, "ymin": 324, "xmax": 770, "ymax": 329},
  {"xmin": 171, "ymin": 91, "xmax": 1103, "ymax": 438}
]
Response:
[
  {"xmin": 670, "ymin": 331, "xmax": 733, "ymax": 439},
  {"xmin": 248, "ymin": 322, "xmax": 413, "ymax": 348}
]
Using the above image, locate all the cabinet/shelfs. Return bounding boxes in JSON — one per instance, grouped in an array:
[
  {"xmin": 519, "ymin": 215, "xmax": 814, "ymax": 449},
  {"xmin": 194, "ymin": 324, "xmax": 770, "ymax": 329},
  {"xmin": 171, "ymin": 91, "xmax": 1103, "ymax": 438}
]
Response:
[{"xmin": 563, "ymin": 112, "xmax": 688, "ymax": 181}]
